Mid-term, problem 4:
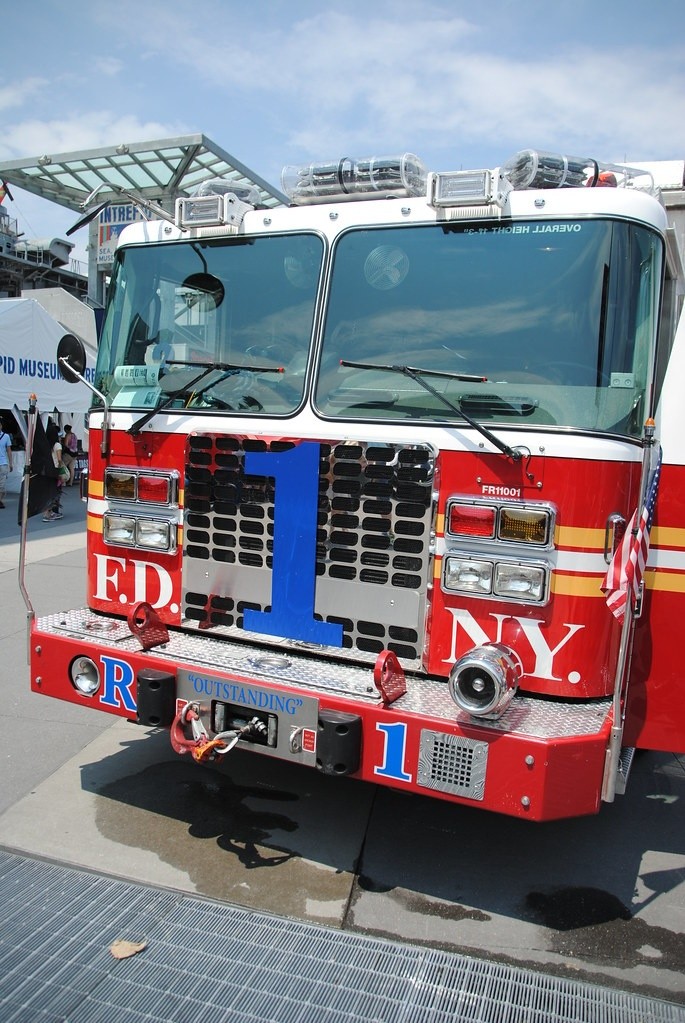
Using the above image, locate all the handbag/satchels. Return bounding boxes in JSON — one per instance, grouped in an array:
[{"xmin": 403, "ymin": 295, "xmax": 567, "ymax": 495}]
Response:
[{"xmin": 57, "ymin": 461, "xmax": 70, "ymax": 483}]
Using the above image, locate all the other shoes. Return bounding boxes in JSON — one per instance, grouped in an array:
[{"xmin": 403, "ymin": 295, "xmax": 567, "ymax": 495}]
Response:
[
  {"xmin": 0, "ymin": 501, "xmax": 5, "ymax": 508},
  {"xmin": 61, "ymin": 483, "xmax": 66, "ymax": 486},
  {"xmin": 42, "ymin": 515, "xmax": 55, "ymax": 521},
  {"xmin": 67, "ymin": 482, "xmax": 72, "ymax": 486},
  {"xmin": 49, "ymin": 512, "xmax": 63, "ymax": 519}
]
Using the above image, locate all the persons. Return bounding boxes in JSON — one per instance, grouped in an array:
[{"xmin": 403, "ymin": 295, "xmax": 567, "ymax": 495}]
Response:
[
  {"xmin": 0, "ymin": 419, "xmax": 13, "ymax": 509},
  {"xmin": 62, "ymin": 424, "xmax": 78, "ymax": 487},
  {"xmin": 43, "ymin": 424, "xmax": 65, "ymax": 522}
]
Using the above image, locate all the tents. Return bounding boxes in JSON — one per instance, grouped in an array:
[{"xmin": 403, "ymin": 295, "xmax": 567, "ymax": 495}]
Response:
[{"xmin": 0, "ymin": 297, "xmax": 97, "ymax": 421}]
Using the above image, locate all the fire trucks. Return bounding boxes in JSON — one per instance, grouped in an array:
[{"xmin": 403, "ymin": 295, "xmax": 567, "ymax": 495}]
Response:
[{"xmin": 0, "ymin": 131, "xmax": 685, "ymax": 827}]
